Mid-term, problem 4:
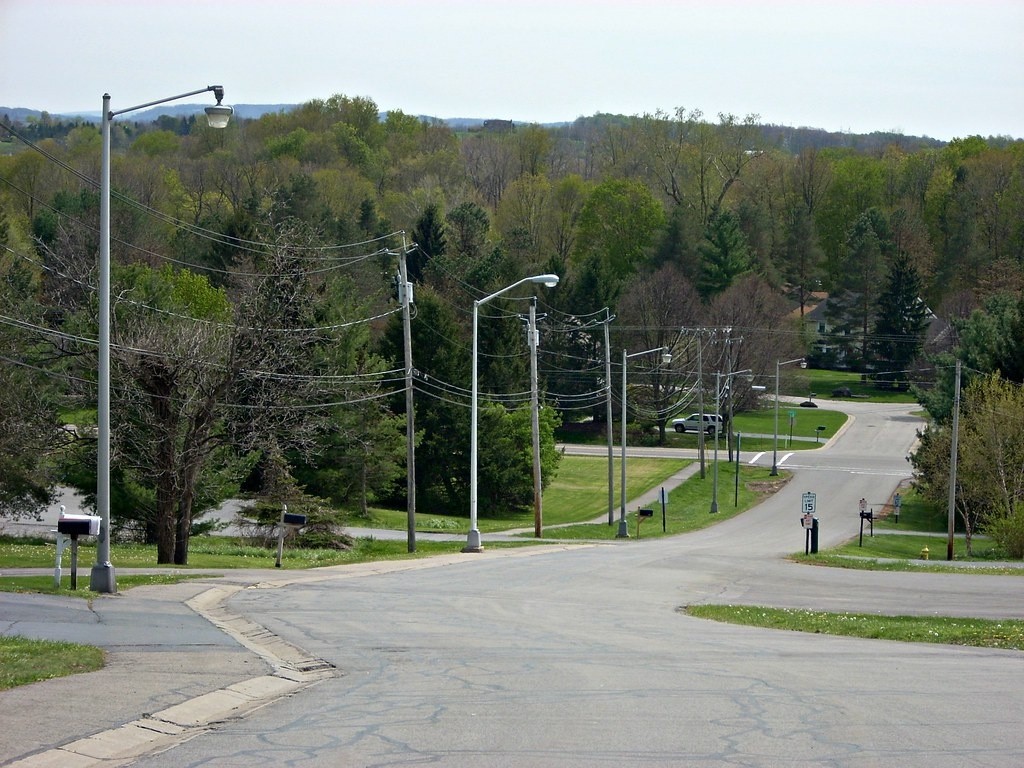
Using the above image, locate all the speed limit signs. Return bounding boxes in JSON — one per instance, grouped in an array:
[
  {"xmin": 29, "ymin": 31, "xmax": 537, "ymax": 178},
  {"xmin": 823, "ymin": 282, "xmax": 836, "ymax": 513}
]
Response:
[{"xmin": 802, "ymin": 492, "xmax": 817, "ymax": 513}]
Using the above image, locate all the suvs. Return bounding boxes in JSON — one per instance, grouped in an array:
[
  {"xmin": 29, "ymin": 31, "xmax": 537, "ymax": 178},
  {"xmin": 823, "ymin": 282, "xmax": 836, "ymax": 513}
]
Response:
[{"xmin": 672, "ymin": 413, "xmax": 723, "ymax": 435}]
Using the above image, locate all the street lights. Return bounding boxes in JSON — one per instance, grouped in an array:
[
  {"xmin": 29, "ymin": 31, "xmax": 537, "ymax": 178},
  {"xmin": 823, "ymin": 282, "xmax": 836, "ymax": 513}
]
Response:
[
  {"xmin": 88, "ymin": 81, "xmax": 236, "ymax": 599},
  {"xmin": 615, "ymin": 346, "xmax": 672, "ymax": 538},
  {"xmin": 708, "ymin": 367, "xmax": 755, "ymax": 516},
  {"xmin": 460, "ymin": 273, "xmax": 560, "ymax": 548},
  {"xmin": 769, "ymin": 356, "xmax": 808, "ymax": 478}
]
